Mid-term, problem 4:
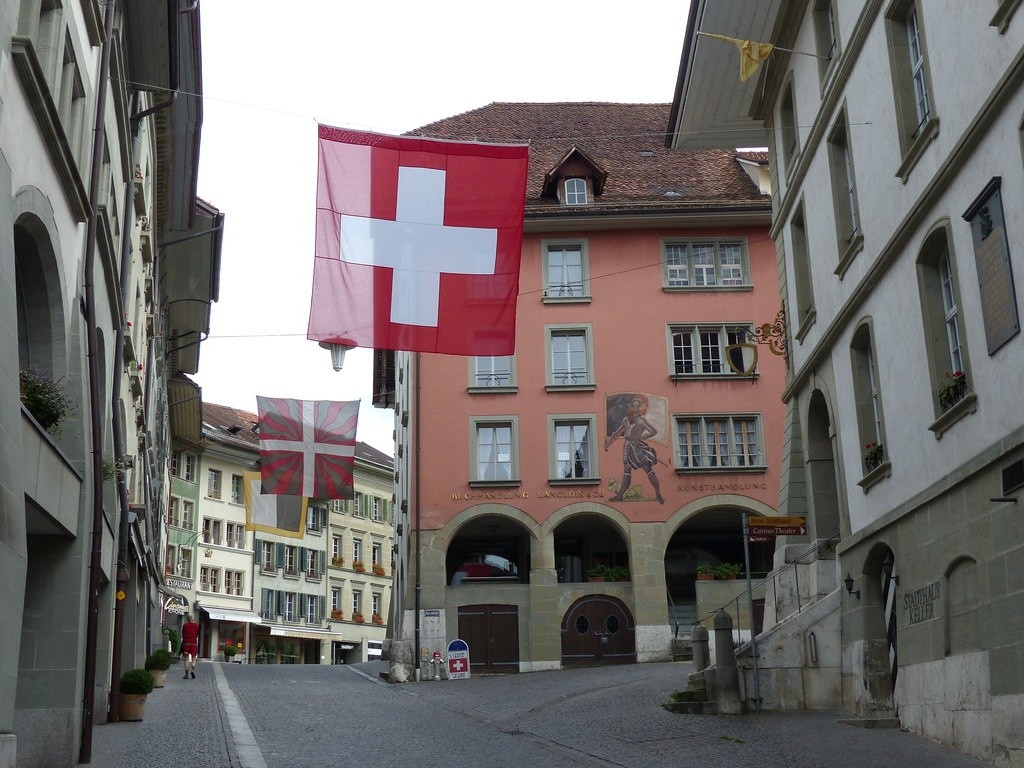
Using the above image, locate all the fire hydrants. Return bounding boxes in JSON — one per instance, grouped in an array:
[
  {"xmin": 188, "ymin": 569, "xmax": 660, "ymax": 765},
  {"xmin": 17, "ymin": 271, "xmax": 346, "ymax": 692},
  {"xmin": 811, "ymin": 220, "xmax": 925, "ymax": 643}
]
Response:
[{"xmin": 432, "ymin": 652, "xmax": 445, "ymax": 680}]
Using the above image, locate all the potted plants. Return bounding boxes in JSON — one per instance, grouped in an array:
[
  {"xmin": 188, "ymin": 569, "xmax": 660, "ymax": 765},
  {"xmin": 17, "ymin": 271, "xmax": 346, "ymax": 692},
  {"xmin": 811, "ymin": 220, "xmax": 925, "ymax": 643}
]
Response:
[
  {"xmin": 713, "ymin": 562, "xmax": 744, "ymax": 580},
  {"xmin": 695, "ymin": 561, "xmax": 715, "ymax": 581},
  {"xmin": 584, "ymin": 564, "xmax": 606, "ymax": 582},
  {"xmin": 167, "ymin": 627, "xmax": 181, "ymax": 665},
  {"xmin": 224, "ymin": 646, "xmax": 238, "ymax": 663},
  {"xmin": 144, "ymin": 649, "xmax": 172, "ymax": 688},
  {"xmin": 119, "ymin": 668, "xmax": 155, "ymax": 723}
]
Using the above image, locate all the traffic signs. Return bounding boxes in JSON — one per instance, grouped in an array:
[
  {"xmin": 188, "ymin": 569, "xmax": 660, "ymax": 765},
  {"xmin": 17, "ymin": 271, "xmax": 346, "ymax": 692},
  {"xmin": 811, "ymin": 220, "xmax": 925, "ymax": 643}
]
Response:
[{"xmin": 747, "ymin": 516, "xmax": 808, "ymax": 543}]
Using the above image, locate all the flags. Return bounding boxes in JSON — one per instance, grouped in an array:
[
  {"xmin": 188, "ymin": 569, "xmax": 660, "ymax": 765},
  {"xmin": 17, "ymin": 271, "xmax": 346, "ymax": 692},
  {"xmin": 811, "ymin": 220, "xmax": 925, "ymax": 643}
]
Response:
[
  {"xmin": 243, "ymin": 471, "xmax": 308, "ymax": 540},
  {"xmin": 256, "ymin": 395, "xmax": 361, "ymax": 500},
  {"xmin": 305, "ymin": 123, "xmax": 528, "ymax": 357}
]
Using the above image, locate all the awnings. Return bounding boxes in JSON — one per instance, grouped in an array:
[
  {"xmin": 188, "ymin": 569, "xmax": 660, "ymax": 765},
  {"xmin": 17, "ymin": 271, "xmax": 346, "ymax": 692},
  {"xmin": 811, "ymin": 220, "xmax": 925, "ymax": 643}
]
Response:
[
  {"xmin": 158, "ymin": 197, "xmax": 225, "ymax": 375},
  {"xmin": 252, "ymin": 621, "xmax": 343, "ymax": 641},
  {"xmin": 199, "ymin": 604, "xmax": 261, "ymax": 623},
  {"xmin": 167, "ymin": 372, "xmax": 202, "ymax": 453}
]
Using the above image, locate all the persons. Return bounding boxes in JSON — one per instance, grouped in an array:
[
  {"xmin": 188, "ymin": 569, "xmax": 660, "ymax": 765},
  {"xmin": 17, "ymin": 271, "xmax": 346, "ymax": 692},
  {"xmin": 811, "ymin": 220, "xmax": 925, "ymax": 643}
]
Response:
[{"xmin": 179, "ymin": 615, "xmax": 199, "ymax": 679}]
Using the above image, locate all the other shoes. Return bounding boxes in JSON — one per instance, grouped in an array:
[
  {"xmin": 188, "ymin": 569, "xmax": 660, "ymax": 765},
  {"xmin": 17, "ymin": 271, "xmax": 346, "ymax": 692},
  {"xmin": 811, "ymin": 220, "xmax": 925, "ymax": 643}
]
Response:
[
  {"xmin": 191, "ymin": 672, "xmax": 196, "ymax": 678},
  {"xmin": 183, "ymin": 675, "xmax": 188, "ymax": 679}
]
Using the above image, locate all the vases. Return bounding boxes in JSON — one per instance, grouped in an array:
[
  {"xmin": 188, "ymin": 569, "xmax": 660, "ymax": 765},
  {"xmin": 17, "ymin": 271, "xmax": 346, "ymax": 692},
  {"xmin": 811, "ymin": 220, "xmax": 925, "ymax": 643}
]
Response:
[
  {"xmin": 20, "ymin": 390, "xmax": 62, "ymax": 433},
  {"xmin": 864, "ymin": 444, "xmax": 884, "ymax": 474},
  {"xmin": 939, "ymin": 374, "xmax": 967, "ymax": 415}
]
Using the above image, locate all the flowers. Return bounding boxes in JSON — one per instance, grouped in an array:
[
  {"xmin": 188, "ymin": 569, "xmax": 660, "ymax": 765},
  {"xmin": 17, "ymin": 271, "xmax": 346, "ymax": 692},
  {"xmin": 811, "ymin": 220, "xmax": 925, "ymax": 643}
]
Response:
[
  {"xmin": 864, "ymin": 440, "xmax": 882, "ymax": 460},
  {"xmin": 20, "ymin": 360, "xmax": 80, "ymax": 441},
  {"xmin": 938, "ymin": 369, "xmax": 965, "ymax": 396}
]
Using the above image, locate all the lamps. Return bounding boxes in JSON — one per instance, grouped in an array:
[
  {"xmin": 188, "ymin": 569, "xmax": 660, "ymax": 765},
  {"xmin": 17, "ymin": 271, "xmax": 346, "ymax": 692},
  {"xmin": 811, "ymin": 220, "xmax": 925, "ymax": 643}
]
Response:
[
  {"xmin": 882, "ymin": 552, "xmax": 900, "ymax": 586},
  {"xmin": 844, "ymin": 572, "xmax": 860, "ymax": 601}
]
